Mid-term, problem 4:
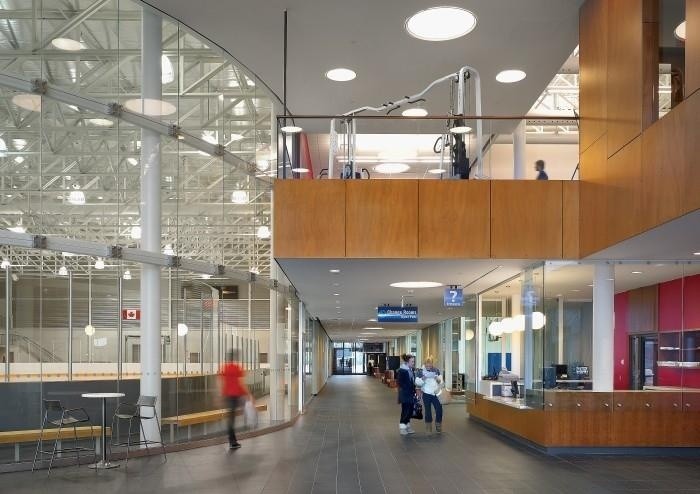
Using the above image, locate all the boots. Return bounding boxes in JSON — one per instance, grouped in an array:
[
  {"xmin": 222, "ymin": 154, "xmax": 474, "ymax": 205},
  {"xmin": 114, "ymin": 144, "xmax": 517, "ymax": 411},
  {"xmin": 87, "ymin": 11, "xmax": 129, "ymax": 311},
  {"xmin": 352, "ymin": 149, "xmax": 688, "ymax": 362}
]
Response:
[
  {"xmin": 434, "ymin": 421, "xmax": 442, "ymax": 434},
  {"xmin": 425, "ymin": 421, "xmax": 433, "ymax": 437},
  {"xmin": 398, "ymin": 423, "xmax": 409, "ymax": 436},
  {"xmin": 405, "ymin": 423, "xmax": 416, "ymax": 434}
]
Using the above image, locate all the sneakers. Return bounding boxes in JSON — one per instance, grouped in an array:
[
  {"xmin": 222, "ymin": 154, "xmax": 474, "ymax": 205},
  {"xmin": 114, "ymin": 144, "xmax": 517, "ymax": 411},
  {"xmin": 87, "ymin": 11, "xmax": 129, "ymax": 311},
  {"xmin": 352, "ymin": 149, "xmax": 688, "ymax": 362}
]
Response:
[{"xmin": 228, "ymin": 442, "xmax": 242, "ymax": 449}]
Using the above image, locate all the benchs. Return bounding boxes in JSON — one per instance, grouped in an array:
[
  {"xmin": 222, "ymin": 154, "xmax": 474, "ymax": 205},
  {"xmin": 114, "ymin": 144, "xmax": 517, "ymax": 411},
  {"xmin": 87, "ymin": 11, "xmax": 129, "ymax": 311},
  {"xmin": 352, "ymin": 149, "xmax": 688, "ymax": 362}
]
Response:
[
  {"xmin": 0, "ymin": 423, "xmax": 114, "ymax": 461},
  {"xmin": 161, "ymin": 403, "xmax": 273, "ymax": 443}
]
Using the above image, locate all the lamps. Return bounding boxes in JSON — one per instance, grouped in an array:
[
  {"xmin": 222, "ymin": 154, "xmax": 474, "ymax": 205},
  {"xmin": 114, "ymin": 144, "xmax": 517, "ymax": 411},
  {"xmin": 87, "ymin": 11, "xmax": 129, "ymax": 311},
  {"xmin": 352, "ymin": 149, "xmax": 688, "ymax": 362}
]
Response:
[
  {"xmin": 1, "ymin": 159, "xmax": 270, "ymax": 336},
  {"xmin": 485, "ymin": 273, "xmax": 548, "ymax": 337}
]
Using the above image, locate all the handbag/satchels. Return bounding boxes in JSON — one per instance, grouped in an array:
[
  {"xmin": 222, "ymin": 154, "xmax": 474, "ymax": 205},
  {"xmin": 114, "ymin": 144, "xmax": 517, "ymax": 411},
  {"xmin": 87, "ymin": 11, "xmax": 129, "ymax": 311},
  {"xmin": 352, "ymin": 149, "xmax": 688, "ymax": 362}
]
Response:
[{"xmin": 412, "ymin": 395, "xmax": 424, "ymax": 419}]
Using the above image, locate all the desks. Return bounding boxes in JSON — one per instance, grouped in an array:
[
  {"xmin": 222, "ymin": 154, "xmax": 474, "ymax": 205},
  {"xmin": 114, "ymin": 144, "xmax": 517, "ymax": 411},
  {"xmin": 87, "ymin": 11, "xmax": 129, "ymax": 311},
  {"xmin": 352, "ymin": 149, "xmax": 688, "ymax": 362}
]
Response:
[{"xmin": 77, "ymin": 391, "xmax": 130, "ymax": 473}]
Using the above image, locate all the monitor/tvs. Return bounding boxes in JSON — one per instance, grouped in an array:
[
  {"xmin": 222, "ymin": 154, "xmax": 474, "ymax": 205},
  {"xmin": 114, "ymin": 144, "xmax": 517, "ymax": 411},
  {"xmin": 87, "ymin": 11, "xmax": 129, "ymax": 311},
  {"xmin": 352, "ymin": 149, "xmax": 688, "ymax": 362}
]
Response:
[
  {"xmin": 552, "ymin": 364, "xmax": 567, "ymax": 378},
  {"xmin": 511, "ymin": 380, "xmax": 519, "ymax": 394},
  {"xmin": 543, "ymin": 367, "xmax": 556, "ymax": 387},
  {"xmin": 576, "ymin": 366, "xmax": 590, "ymax": 377}
]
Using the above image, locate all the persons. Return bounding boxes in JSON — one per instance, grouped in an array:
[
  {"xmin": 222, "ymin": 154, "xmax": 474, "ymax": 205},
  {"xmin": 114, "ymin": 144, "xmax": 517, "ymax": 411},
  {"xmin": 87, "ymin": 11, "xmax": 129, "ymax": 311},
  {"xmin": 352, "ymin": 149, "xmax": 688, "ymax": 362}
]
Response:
[
  {"xmin": 395, "ymin": 353, "xmax": 417, "ymax": 435},
  {"xmin": 535, "ymin": 160, "xmax": 548, "ymax": 180},
  {"xmin": 340, "ymin": 357, "xmax": 352, "ymax": 369},
  {"xmin": 368, "ymin": 363, "xmax": 374, "ymax": 376},
  {"xmin": 415, "ymin": 356, "xmax": 444, "ymax": 436},
  {"xmin": 218, "ymin": 347, "xmax": 256, "ymax": 449}
]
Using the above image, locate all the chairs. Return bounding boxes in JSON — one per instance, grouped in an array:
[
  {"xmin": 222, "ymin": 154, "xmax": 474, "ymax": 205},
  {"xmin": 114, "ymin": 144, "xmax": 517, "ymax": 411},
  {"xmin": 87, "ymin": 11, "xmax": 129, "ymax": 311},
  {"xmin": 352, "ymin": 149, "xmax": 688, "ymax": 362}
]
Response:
[
  {"xmin": 27, "ymin": 396, "xmax": 99, "ymax": 475},
  {"xmin": 108, "ymin": 392, "xmax": 171, "ymax": 470}
]
